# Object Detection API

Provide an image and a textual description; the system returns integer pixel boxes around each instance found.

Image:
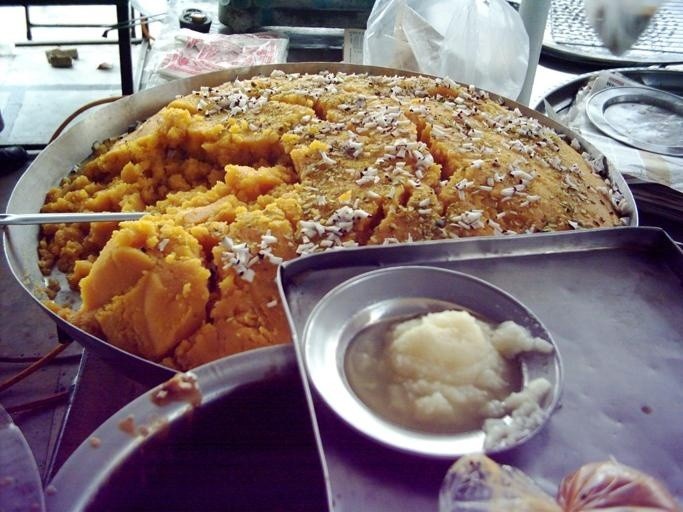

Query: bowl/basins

[42,346,316,512]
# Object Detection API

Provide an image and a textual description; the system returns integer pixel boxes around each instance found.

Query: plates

[303,264,561,458]
[586,86,683,157]
[2,60,639,373]
